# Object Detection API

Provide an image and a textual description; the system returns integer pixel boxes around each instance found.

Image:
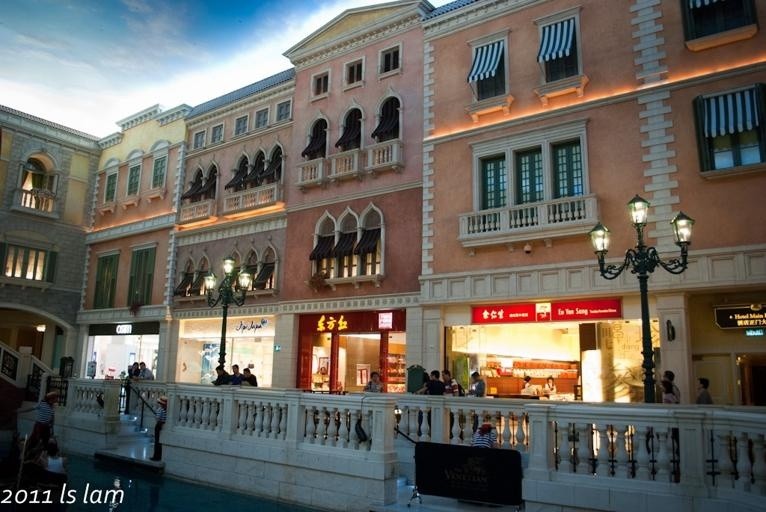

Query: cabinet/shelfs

[389,354,407,384]
[481,354,578,394]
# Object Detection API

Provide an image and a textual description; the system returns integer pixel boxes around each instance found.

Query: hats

[158,396,168,405]
[46,392,58,401]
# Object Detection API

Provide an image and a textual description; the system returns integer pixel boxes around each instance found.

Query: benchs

[407,441,526,512]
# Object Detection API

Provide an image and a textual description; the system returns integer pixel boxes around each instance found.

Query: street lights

[586,194,696,404]
[201,254,253,370]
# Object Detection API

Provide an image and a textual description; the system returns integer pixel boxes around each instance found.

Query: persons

[241,367,258,387]
[363,371,385,393]
[694,377,714,405]
[211,365,231,386]
[659,381,679,404]
[469,423,498,449]
[132,362,155,381]
[468,371,485,433]
[124,362,141,415]
[228,364,245,385]
[545,377,557,394]
[411,368,465,442]
[149,395,168,462]
[662,371,679,396]
[521,376,531,389]
[16,390,59,464]
